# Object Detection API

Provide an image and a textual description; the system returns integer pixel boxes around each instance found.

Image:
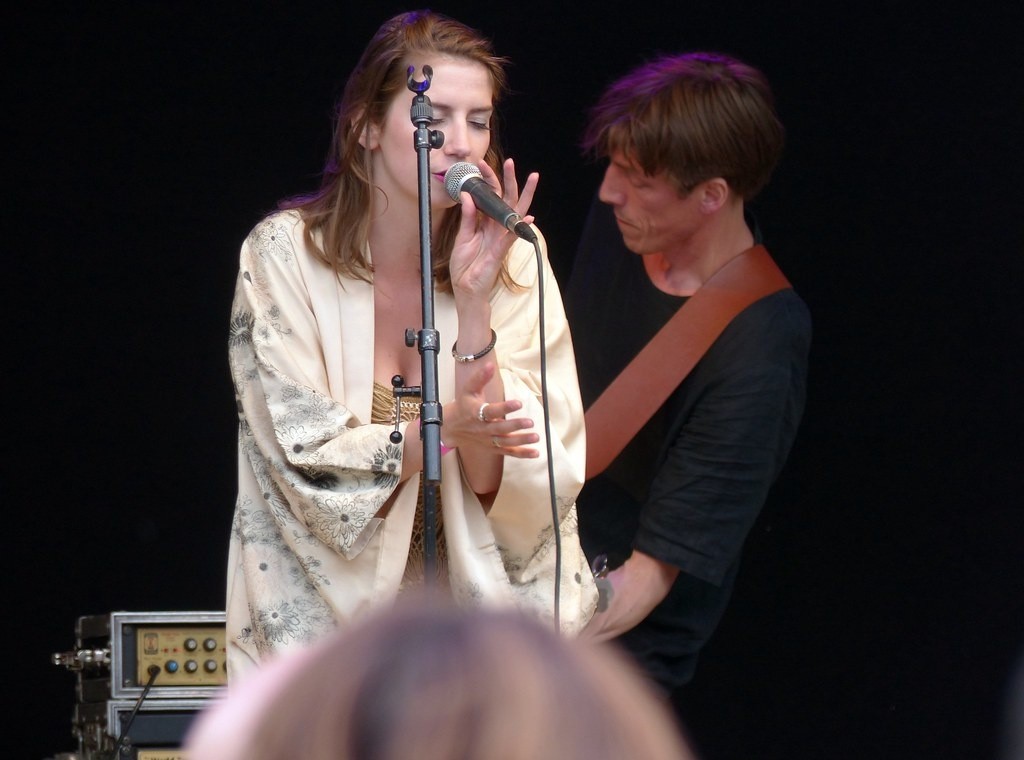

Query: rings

[478,403,497,424]
[491,436,503,448]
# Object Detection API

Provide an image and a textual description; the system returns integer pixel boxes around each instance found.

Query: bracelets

[451,328,497,363]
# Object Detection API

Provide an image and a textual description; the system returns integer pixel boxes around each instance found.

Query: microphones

[443,163,536,243]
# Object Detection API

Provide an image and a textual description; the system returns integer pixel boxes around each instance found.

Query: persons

[225,11,598,703]
[560,53,814,706]
[186,593,696,759]
